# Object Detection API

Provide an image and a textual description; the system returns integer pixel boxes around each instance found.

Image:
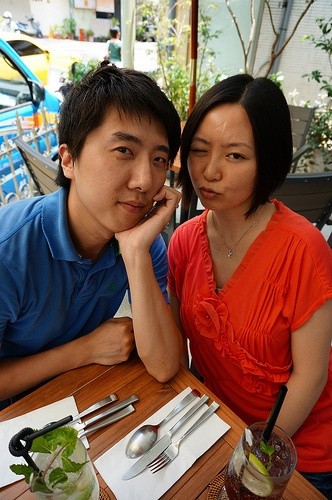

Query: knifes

[75,394,139,431]
[121,394,209,481]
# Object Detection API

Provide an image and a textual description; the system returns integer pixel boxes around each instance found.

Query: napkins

[93,387,231,500]
[0,396,90,489]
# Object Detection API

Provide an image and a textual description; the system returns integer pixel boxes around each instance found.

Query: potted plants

[63,18,76,40]
[86,30,94,42]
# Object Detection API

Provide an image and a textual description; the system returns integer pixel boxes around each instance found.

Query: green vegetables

[259,441,275,462]
[9,426,93,500]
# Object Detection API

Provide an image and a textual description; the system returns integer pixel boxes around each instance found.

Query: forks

[147,400,219,476]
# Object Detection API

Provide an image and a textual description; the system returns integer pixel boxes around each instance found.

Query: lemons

[234,450,273,497]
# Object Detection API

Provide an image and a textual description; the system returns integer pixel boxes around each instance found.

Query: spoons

[125,389,201,458]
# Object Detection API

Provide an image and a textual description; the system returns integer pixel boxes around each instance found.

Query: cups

[28,437,99,500]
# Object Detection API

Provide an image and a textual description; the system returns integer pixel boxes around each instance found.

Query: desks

[0,335,325,500]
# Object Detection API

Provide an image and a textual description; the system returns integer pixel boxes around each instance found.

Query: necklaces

[206,199,264,256]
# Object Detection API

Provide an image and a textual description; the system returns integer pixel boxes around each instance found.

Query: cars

[0,34,64,207]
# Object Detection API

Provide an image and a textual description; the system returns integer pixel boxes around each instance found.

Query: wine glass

[219,421,298,500]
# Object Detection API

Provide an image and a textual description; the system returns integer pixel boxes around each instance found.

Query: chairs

[15,141,63,198]
[282,170,332,231]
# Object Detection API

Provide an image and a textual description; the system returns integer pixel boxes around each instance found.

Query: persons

[108,25,122,71]
[0,64,182,415]
[0,9,20,34]
[167,73,332,500]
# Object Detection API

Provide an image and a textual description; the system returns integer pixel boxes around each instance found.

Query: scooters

[14,16,43,38]
